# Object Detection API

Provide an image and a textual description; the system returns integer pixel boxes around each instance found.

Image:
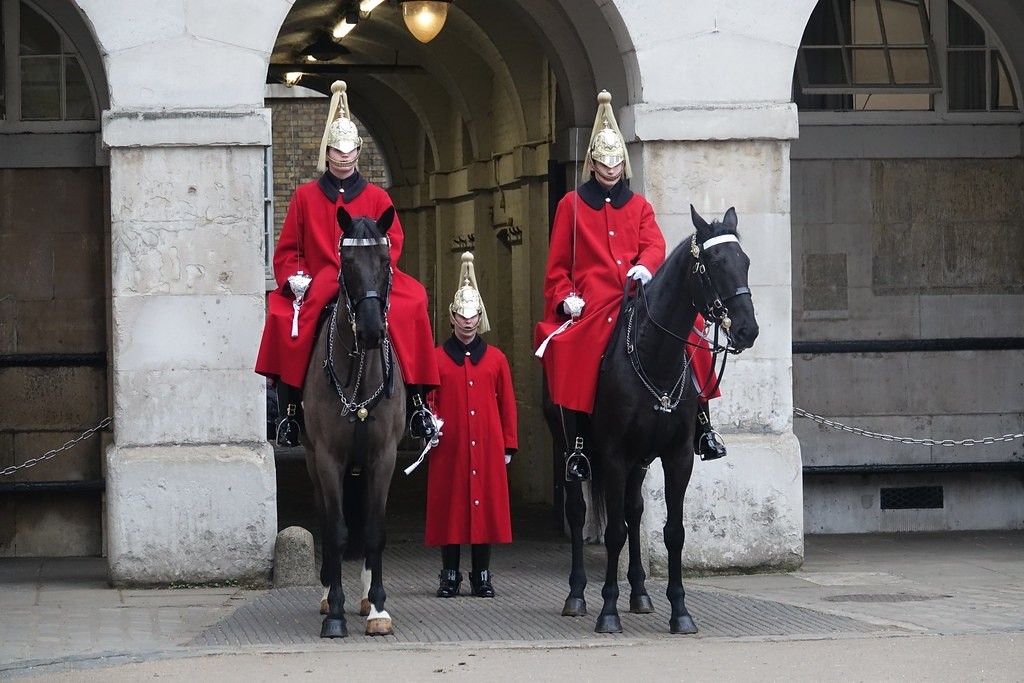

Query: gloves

[505,454,512,464]
[564,294,585,317]
[430,430,442,447]
[626,264,652,286]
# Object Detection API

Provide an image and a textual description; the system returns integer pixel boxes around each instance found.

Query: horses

[541,203,760,635]
[302,205,408,638]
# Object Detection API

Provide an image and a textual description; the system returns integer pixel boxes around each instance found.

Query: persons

[254,80,441,447]
[532,88,727,482]
[428,251,516,598]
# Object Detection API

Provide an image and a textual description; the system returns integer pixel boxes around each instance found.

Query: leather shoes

[278,413,298,445]
[694,425,727,459]
[437,570,464,597]
[408,401,434,435]
[471,570,495,597]
[563,441,591,478]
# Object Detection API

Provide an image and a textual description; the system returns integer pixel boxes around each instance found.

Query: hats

[448,252,491,334]
[581,88,632,184]
[317,80,362,175]
